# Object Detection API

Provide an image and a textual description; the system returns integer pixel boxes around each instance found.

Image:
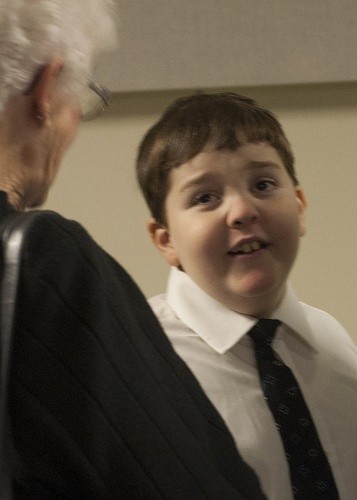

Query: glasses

[80,79,113,122]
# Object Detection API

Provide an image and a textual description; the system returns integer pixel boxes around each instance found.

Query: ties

[247,319,342,500]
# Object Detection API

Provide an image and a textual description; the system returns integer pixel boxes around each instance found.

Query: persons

[0,1,268,500]
[136,91,357,500]
